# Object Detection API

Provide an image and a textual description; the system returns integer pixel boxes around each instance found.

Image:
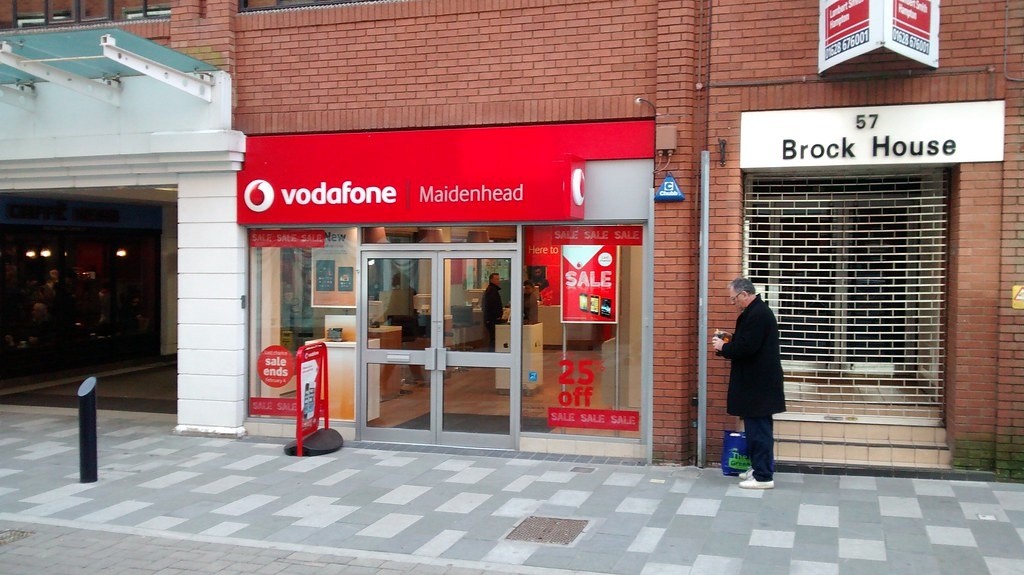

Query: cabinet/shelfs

[304,305,603,423]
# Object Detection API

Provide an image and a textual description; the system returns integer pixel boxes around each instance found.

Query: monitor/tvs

[369,300,384,329]
[467,291,483,308]
[413,296,432,314]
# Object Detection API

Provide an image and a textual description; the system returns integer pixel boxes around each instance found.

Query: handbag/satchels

[721,430,775,476]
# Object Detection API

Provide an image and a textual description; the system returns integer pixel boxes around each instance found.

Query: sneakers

[738,476,774,489]
[738,466,755,478]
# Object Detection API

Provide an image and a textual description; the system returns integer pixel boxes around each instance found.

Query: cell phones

[579,293,612,318]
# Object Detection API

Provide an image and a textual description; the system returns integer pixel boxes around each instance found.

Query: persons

[524,280,538,325]
[482,273,503,352]
[712,278,787,489]
[533,267,546,286]
[537,279,553,306]
[370,273,425,399]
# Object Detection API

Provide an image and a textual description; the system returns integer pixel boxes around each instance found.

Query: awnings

[0,30,219,81]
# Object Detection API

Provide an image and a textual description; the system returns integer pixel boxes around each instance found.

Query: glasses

[731,293,741,302]
